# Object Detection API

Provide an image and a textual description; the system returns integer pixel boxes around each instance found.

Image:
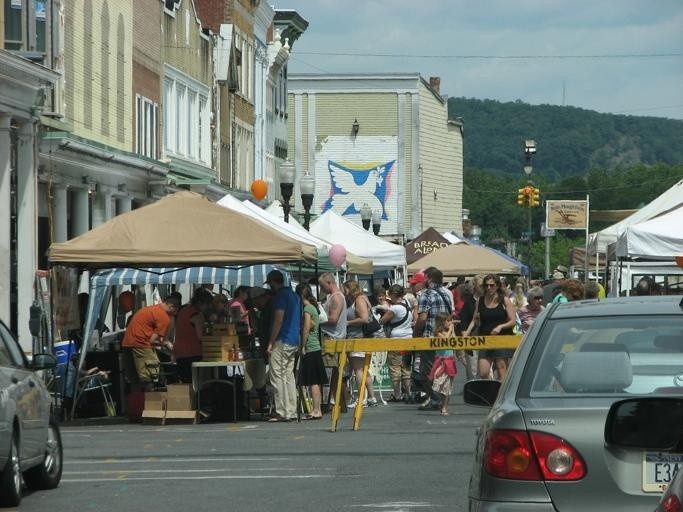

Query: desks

[191,358,270,423]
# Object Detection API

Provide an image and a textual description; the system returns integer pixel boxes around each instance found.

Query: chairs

[67,362,117,420]
[559,351,634,393]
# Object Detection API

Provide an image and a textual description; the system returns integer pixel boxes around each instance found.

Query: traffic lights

[522,188,531,209]
[533,188,540,208]
[517,186,526,208]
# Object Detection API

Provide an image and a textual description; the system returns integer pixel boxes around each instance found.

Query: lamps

[352,116,360,139]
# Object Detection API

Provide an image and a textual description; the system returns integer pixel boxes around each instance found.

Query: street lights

[280,152,314,290]
[520,137,539,282]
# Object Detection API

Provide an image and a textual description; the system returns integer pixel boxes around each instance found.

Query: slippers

[439,409,448,416]
[387,392,428,402]
[267,414,292,423]
[300,413,322,421]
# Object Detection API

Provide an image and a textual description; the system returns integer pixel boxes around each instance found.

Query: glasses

[534,295,545,301]
[484,283,497,288]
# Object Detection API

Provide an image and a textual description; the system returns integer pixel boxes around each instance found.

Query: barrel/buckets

[43,339,78,393]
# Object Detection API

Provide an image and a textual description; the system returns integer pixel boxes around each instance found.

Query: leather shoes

[419,401,441,411]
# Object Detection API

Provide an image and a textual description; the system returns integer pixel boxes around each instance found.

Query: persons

[637,275,667,296]
[65,291,112,388]
[122,268,605,423]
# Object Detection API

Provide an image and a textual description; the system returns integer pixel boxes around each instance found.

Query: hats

[409,274,424,284]
[263,270,284,284]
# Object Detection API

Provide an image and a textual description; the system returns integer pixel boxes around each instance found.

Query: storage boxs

[144,383,192,411]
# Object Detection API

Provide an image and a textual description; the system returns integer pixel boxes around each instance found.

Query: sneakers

[347,398,370,410]
[366,397,379,407]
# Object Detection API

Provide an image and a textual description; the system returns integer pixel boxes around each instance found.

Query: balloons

[327,245,346,267]
[250,180,268,202]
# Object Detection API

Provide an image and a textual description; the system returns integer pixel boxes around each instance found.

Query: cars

[1,321,63,507]
[461,291,683,512]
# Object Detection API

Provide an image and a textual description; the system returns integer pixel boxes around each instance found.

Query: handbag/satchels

[317,303,329,325]
[362,317,381,337]
